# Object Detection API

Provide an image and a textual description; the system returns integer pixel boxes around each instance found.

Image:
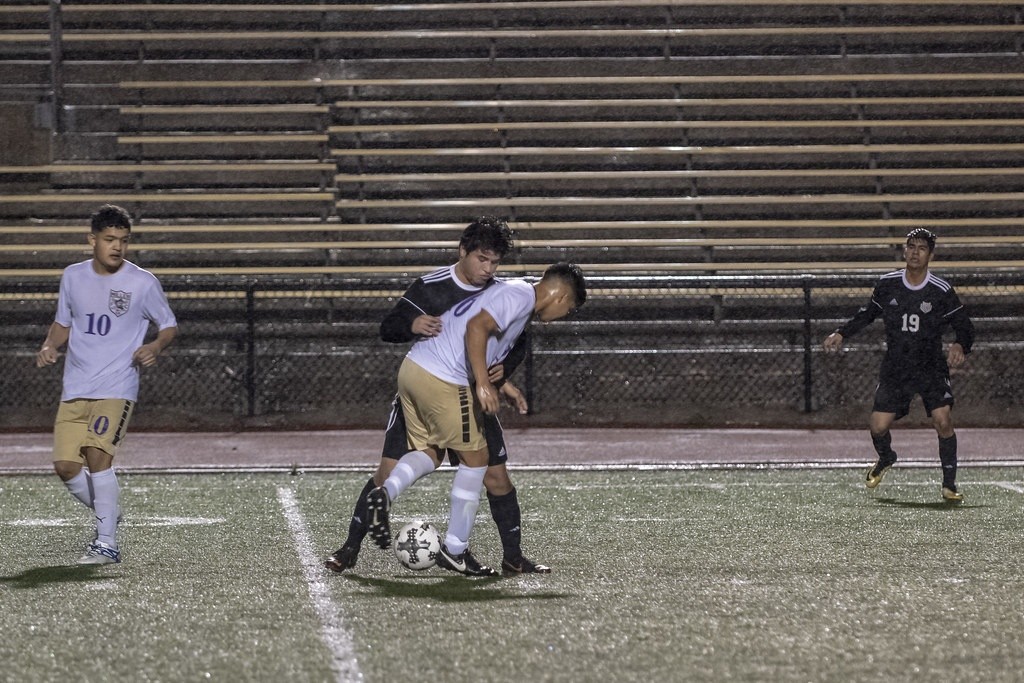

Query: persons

[323,212,552,576]
[365,263,587,577]
[34,204,181,566]
[822,228,977,501]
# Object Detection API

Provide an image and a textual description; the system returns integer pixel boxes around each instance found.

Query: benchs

[1,1,1024,419]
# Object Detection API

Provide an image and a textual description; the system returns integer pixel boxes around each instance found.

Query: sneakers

[368,486,392,551]
[436,541,498,576]
[326,543,360,572]
[502,556,552,574]
[76,541,121,565]
[868,449,897,488]
[942,485,962,500]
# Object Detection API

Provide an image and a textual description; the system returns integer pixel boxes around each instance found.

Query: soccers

[393,518,441,571]
